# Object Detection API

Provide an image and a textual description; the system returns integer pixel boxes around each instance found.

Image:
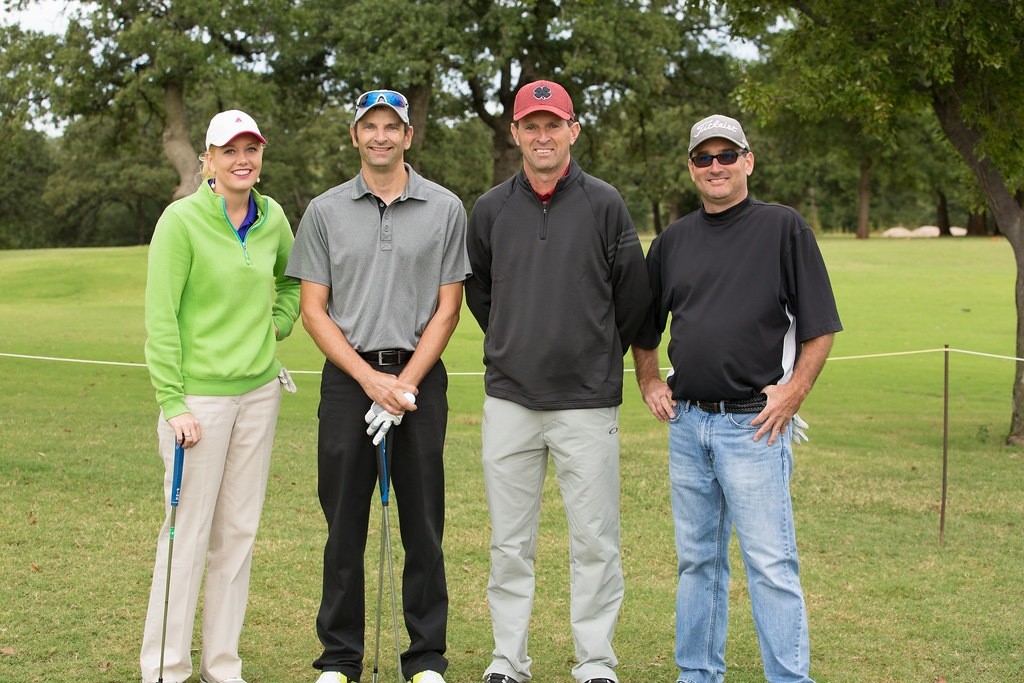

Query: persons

[282,88,473,682]
[139,109,302,683]
[462,78,653,682]
[628,112,847,682]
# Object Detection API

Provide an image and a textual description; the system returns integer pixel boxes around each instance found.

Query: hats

[687,114,750,154]
[513,79,573,122]
[352,88,412,126]
[205,109,266,147]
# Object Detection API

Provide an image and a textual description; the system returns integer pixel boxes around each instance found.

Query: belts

[357,350,417,367]
[689,395,768,414]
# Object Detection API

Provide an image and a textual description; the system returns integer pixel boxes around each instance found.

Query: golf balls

[404,392,416,405]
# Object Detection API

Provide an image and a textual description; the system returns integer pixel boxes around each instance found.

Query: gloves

[364,393,418,445]
[278,367,298,394]
[792,413,810,445]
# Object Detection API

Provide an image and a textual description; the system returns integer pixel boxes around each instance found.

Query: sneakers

[407,670,447,683]
[484,673,519,683]
[314,671,358,683]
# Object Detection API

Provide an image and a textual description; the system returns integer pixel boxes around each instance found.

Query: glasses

[690,148,745,168]
[356,90,407,108]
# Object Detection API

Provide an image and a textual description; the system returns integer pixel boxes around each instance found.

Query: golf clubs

[156,433,185,683]
[373,425,395,683]
[374,427,404,683]
